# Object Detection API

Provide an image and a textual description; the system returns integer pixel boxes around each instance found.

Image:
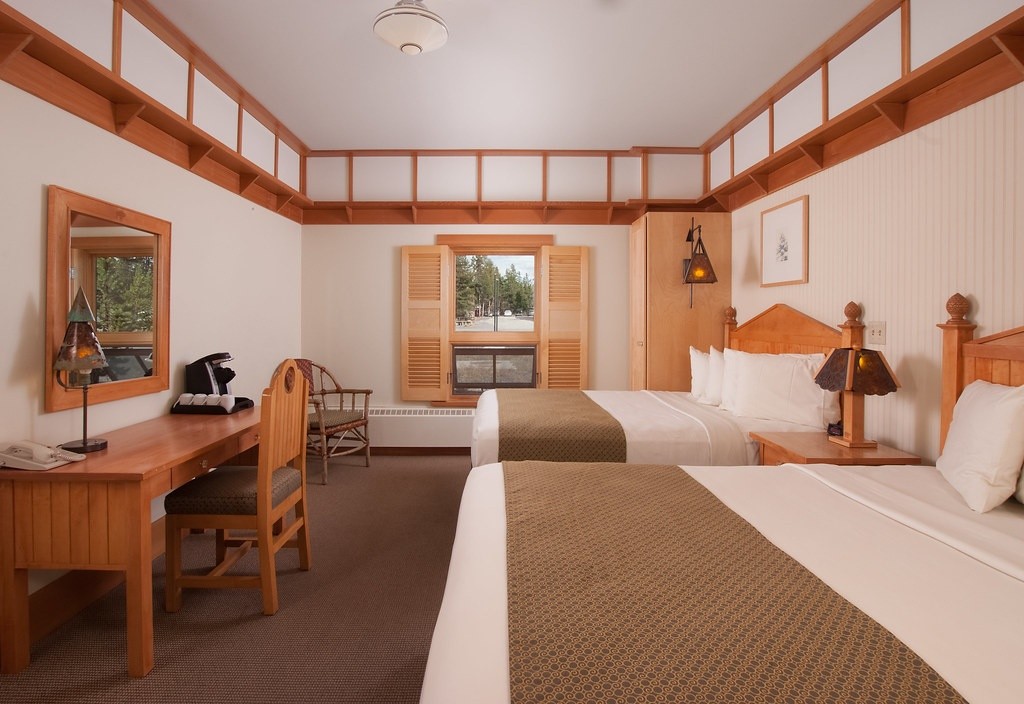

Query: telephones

[0,440,78,471]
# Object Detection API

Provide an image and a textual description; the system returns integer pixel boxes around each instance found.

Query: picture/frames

[760,194,809,287]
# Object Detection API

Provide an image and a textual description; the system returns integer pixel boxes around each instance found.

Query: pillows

[935,378,1024,515]
[685,345,842,430]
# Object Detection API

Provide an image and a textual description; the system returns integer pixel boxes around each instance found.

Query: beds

[417,293,1024,703]
[471,301,866,466]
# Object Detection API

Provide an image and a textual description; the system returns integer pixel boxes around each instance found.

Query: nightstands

[749,430,921,466]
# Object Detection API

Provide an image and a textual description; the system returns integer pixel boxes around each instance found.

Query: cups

[188,394,207,405]
[201,394,220,405]
[173,393,194,408]
[216,393,235,413]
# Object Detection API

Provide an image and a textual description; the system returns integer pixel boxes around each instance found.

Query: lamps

[51,288,112,453]
[812,348,901,448]
[682,218,719,308]
[373,0,450,56]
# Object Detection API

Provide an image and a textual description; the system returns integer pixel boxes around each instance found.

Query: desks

[0,406,287,676]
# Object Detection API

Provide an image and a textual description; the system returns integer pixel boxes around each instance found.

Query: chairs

[274,358,374,486]
[165,358,314,616]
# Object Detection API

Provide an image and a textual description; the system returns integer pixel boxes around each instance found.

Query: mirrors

[43,184,172,410]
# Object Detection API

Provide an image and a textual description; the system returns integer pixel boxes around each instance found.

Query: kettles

[185,352,236,395]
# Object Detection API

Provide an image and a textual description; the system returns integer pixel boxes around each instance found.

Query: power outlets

[867,321,885,345]
